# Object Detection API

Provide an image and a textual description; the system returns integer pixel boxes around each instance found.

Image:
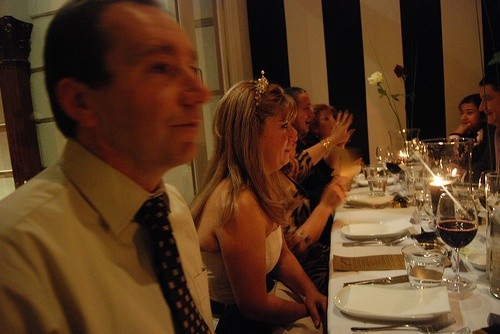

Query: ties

[132,193,212,334]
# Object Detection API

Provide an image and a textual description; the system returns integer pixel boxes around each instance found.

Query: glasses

[97,59,204,91]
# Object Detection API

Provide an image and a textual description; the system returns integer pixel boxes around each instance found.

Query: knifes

[343,275,409,288]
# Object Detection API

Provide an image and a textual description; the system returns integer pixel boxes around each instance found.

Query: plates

[345,194,394,206]
[466,248,487,270]
[334,285,442,322]
[357,177,396,186]
[341,222,405,239]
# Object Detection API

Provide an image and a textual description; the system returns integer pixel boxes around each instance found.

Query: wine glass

[375,145,392,176]
[386,155,404,185]
[435,193,480,294]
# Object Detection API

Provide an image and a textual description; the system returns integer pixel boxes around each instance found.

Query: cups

[368,176,388,196]
[388,128,420,166]
[478,171,491,208]
[484,170,500,299]
[403,243,448,288]
[419,138,472,204]
[362,164,376,181]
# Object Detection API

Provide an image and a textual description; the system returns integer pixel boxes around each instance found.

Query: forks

[455,326,471,334]
[350,317,456,334]
[342,233,408,247]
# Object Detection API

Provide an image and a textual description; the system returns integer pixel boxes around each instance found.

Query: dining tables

[326,177,500,334]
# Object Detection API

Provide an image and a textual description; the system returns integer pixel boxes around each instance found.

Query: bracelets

[320,137,335,153]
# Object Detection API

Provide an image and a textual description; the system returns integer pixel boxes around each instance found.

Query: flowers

[367,64,411,149]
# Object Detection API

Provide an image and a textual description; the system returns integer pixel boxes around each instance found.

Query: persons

[188,69,328,334]
[280,85,367,300]
[0,0,214,333]
[448,67,500,194]
